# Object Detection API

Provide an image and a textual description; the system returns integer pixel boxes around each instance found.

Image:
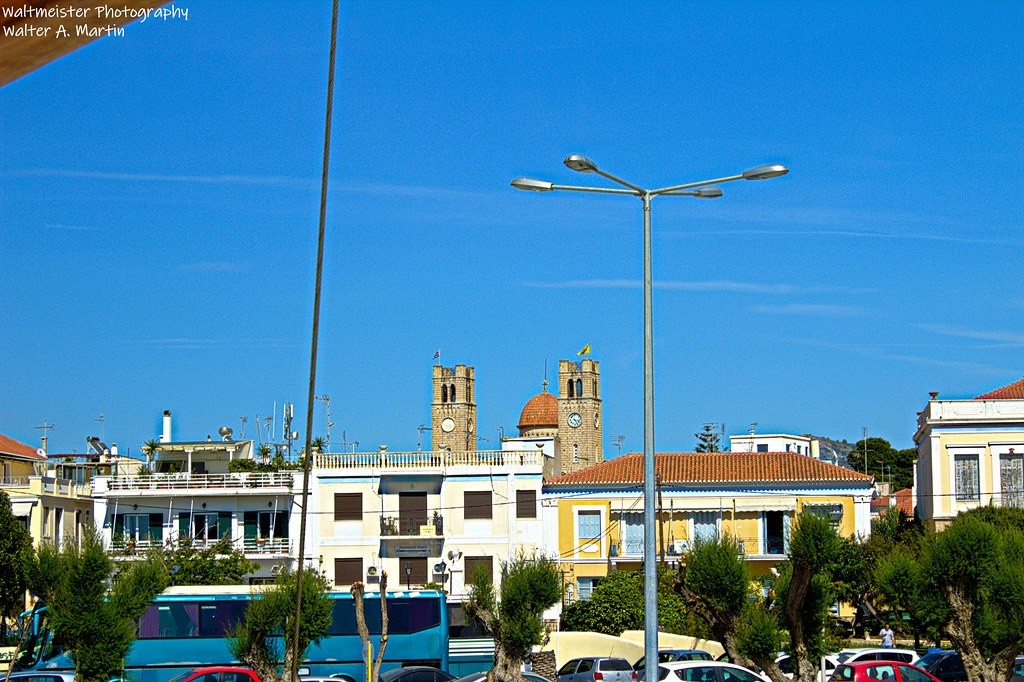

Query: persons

[852,607,865,638]
[879,623,896,649]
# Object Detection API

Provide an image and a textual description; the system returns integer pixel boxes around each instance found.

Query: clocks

[593,412,599,429]
[568,412,582,428]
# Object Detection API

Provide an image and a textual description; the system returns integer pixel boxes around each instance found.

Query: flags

[577,344,590,356]
[433,351,439,360]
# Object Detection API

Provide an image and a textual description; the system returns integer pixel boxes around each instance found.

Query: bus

[5,584,448,682]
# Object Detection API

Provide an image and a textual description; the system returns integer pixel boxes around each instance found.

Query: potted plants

[137,463,273,487]
[128,529,289,553]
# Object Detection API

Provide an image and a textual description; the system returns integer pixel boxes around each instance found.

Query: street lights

[510,152,788,682]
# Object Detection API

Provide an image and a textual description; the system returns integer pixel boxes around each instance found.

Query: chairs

[706,670,714,682]
[580,665,589,672]
[678,656,687,661]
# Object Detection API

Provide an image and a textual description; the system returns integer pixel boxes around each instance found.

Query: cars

[0,650,840,682]
[828,659,941,682]
[844,649,919,665]
[913,650,966,682]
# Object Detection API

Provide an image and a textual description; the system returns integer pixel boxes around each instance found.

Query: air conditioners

[431,561,449,574]
[367,566,383,576]
